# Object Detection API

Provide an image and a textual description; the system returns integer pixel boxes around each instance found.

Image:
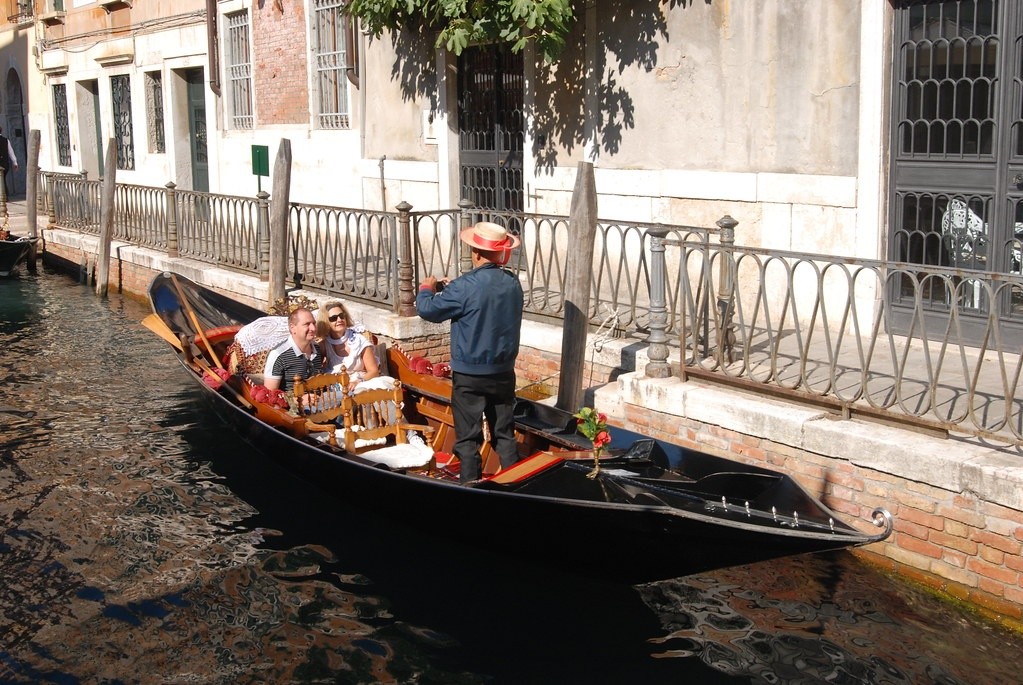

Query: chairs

[292,366,437,479]
[941,198,1023,310]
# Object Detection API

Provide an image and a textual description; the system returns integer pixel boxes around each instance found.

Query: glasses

[328,312,345,322]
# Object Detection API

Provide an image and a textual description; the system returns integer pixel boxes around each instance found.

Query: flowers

[573,406,612,479]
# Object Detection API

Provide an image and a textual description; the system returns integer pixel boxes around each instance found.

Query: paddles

[141,312,254,410]
[170,273,224,370]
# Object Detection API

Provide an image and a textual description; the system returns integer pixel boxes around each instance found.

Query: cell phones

[436,282,444,292]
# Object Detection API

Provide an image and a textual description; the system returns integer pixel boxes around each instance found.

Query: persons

[316,301,424,446]
[416,222,523,483]
[264,308,357,428]
[0,126,19,202]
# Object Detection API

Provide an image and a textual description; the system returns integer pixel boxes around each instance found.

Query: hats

[460,222,520,251]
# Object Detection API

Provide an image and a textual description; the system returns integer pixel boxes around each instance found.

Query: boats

[0,227,42,277]
[144,266,898,605]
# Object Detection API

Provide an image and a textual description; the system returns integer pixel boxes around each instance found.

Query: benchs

[223,314,394,412]
[194,325,243,353]
